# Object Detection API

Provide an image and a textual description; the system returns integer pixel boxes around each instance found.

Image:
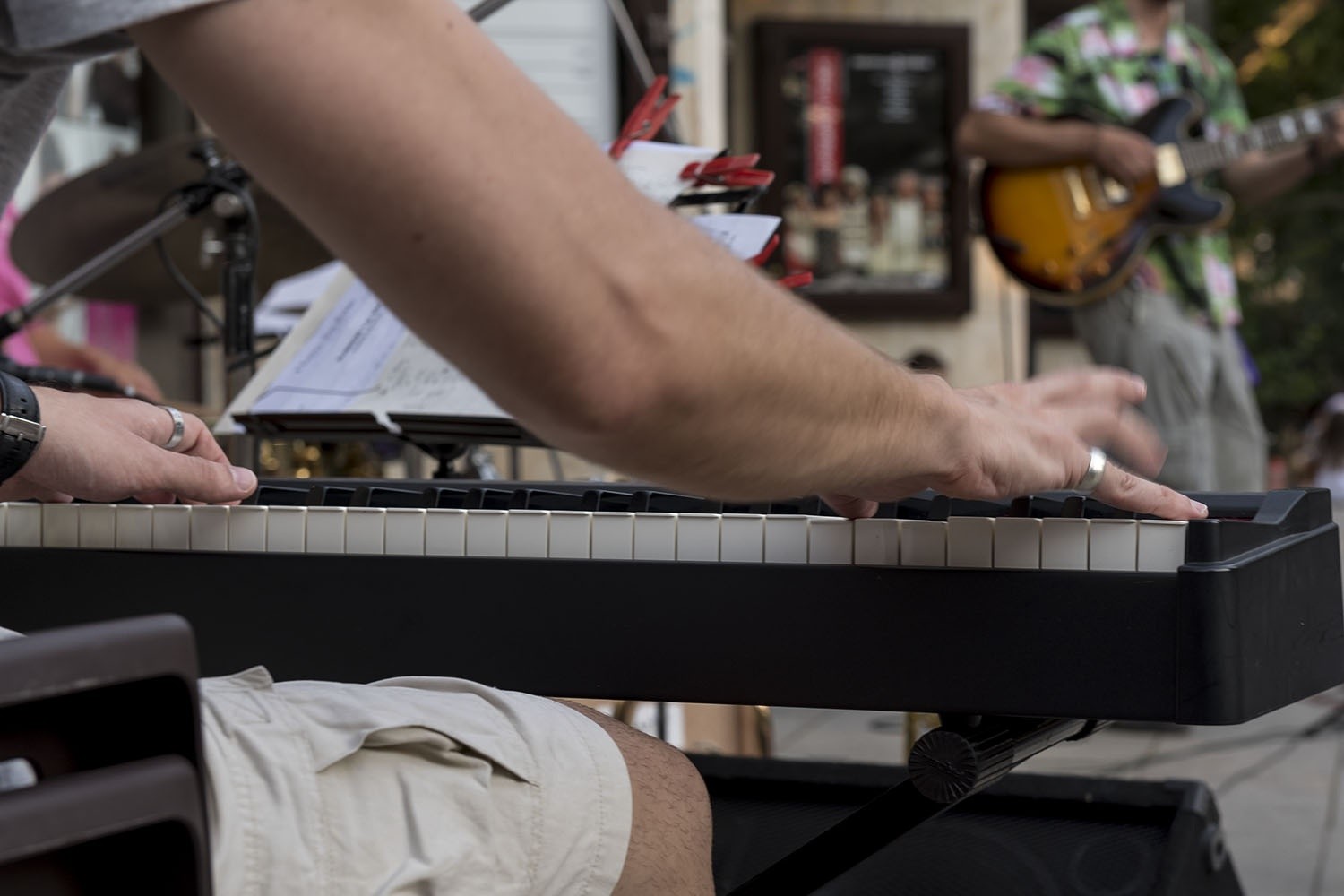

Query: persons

[958,0,1344,494]
[0,0,1207,896]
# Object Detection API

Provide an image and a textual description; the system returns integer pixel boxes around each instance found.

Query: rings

[1074,445,1107,494]
[158,406,185,449]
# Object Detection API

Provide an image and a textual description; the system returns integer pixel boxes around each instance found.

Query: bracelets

[0,371,47,487]
[1307,139,1323,169]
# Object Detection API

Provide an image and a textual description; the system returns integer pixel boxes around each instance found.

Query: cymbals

[9,134,342,307]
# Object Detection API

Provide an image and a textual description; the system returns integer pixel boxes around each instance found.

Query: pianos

[0,477,1344,896]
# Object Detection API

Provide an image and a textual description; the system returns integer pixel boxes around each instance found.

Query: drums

[201,414,379,481]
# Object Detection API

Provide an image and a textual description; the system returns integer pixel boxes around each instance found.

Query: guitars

[968,95,1344,307]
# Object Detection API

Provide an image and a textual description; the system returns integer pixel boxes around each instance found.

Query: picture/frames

[750,21,973,322]
